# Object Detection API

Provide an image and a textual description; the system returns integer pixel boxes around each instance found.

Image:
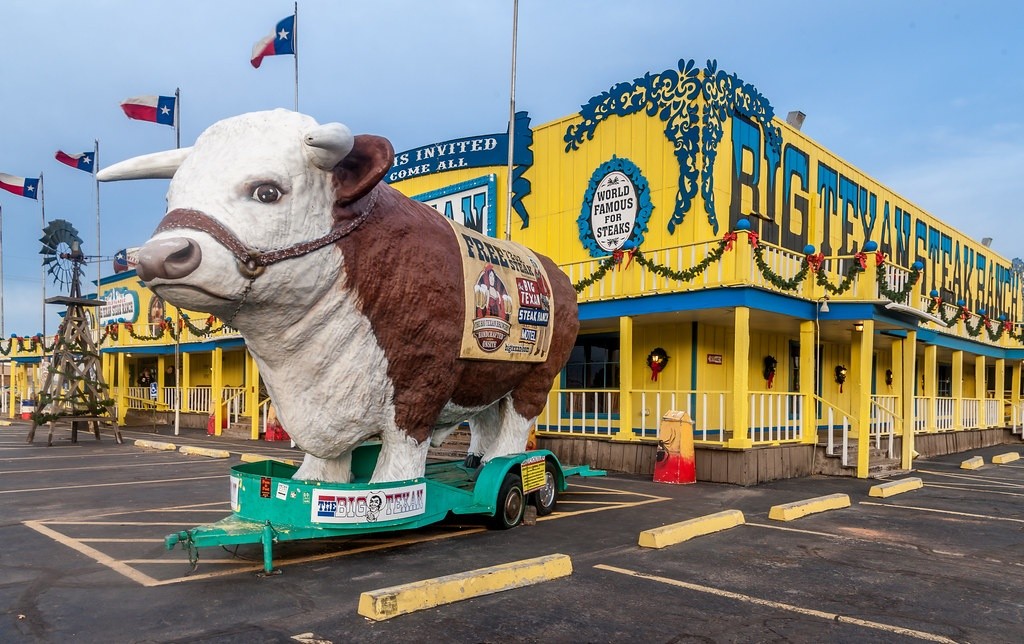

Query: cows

[96,107,580,483]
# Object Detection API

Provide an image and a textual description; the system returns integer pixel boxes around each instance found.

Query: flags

[0,172,39,200]
[251,15,295,70]
[55,150,94,173]
[120,95,175,126]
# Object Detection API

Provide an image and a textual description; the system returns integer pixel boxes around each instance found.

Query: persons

[136,368,155,399]
[164,366,176,409]
